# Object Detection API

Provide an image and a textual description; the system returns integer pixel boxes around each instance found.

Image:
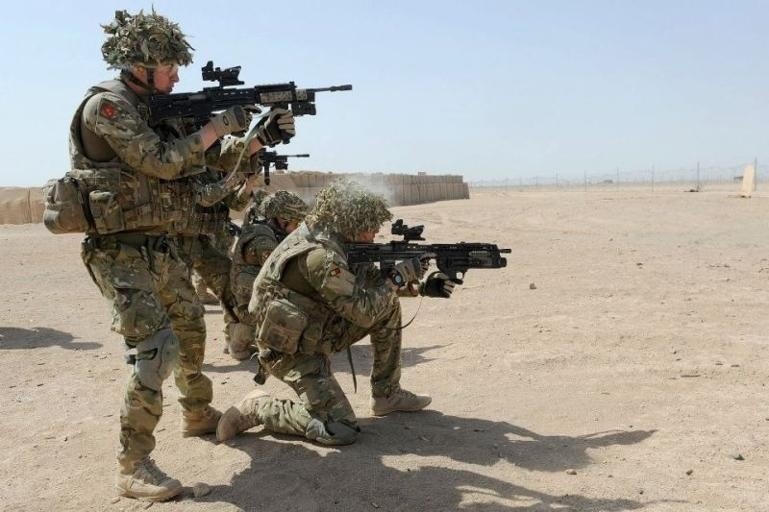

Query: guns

[146,60,353,147]
[258,149,310,183]
[342,219,511,281]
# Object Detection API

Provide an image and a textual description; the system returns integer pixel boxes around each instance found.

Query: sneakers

[183,405,223,438]
[195,287,218,304]
[117,457,183,502]
[370,389,432,416]
[215,389,270,442]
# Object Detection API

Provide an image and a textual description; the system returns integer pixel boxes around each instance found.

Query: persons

[68,10,294,502]
[194,271,218,304]
[216,176,454,445]
[192,173,270,354]
[227,191,310,361]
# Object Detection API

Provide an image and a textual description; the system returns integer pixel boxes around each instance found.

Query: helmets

[310,177,393,233]
[254,189,309,223]
[101,10,195,69]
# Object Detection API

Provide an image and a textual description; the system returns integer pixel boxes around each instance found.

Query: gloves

[418,272,462,297]
[393,253,430,282]
[257,108,295,145]
[211,103,261,137]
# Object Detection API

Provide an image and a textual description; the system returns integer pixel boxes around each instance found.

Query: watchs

[388,272,405,286]
